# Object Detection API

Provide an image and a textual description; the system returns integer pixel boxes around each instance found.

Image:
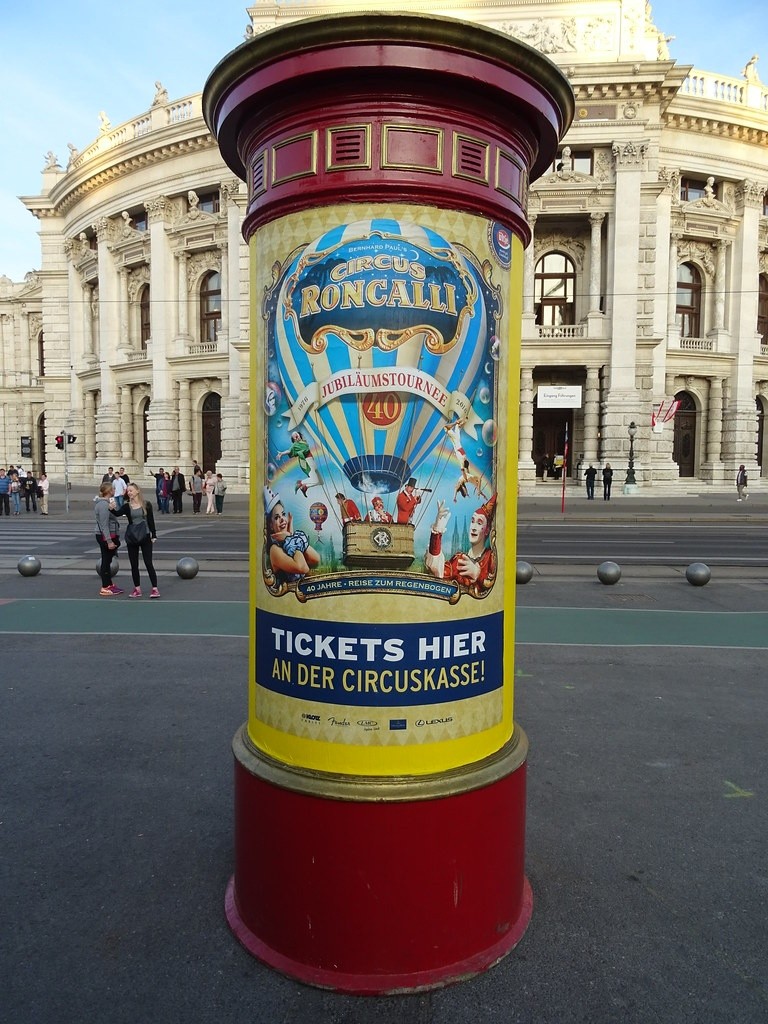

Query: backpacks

[555,455,563,468]
[185,476,196,496]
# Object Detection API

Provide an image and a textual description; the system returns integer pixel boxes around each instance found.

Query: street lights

[623,421,637,484]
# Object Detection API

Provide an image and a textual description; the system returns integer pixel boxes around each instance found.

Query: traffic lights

[66,434,78,444]
[53,435,64,451]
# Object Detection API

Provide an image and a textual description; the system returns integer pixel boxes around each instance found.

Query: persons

[152,82,169,106]
[151,459,227,515]
[584,463,597,500]
[0,465,49,516]
[102,467,130,517]
[80,232,88,245]
[263,417,498,593]
[94,482,125,596]
[67,143,79,160]
[542,451,562,482]
[188,191,199,206]
[595,152,610,181]
[99,112,111,133]
[704,177,715,192]
[562,147,571,163]
[503,4,675,62]
[725,187,735,207]
[108,483,160,597]
[43,151,62,170]
[602,463,613,501]
[736,465,749,502]
[740,55,759,84]
[122,211,133,226]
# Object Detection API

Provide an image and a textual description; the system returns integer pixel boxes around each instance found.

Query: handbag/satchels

[124,521,148,547]
[103,534,122,552]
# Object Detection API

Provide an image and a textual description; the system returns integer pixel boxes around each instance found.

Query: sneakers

[736,499,742,501]
[150,587,161,598]
[745,494,749,500]
[128,586,143,598]
[99,584,124,595]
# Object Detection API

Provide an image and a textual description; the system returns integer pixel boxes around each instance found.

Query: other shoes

[122,514,127,517]
[173,511,177,514]
[608,498,610,500]
[157,509,161,511]
[162,512,164,514]
[13,512,20,515]
[0,514,2,516]
[604,498,606,500]
[542,481,547,482]
[194,512,202,515]
[40,513,48,515]
[6,514,9,516]
[587,497,594,500]
[179,510,182,513]
[166,512,170,514]
[206,512,213,515]
[215,513,222,515]
[553,477,559,480]
